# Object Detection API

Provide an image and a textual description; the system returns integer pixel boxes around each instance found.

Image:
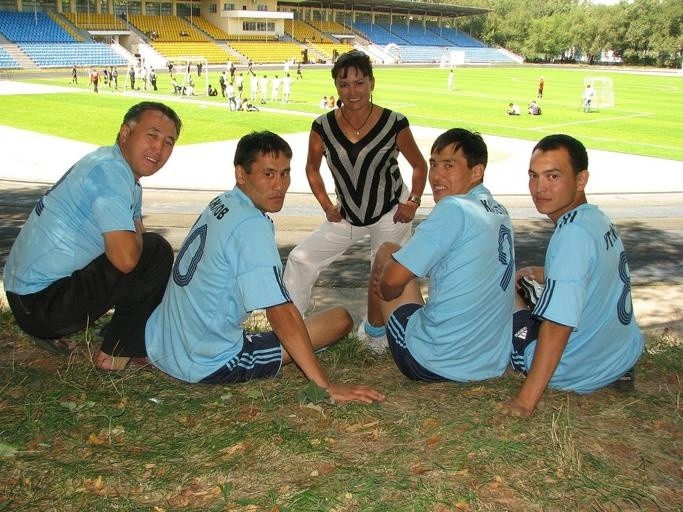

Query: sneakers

[615,368,634,391]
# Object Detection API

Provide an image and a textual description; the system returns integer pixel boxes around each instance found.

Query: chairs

[0,11,523,70]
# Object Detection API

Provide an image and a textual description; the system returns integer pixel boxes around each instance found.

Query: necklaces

[339,104,374,136]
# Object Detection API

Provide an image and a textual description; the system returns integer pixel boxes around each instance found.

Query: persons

[221,60,293,112]
[180,31,190,37]
[363,128,517,383]
[448,70,453,90]
[91,69,100,93]
[173,76,194,96]
[536,76,545,99]
[168,64,173,77]
[208,84,216,96]
[142,128,386,405]
[72,65,78,84]
[281,45,428,355]
[3,100,183,372]
[146,31,159,42]
[526,100,542,116]
[129,66,157,90]
[496,133,645,420]
[284,60,303,79]
[320,96,335,110]
[582,84,595,112]
[104,66,118,90]
[121,11,126,17]
[506,102,522,116]
[197,63,202,77]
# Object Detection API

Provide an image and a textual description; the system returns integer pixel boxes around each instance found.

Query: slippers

[114,356,154,372]
[38,337,71,355]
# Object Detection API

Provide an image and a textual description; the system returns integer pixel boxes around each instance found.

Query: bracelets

[407,194,423,207]
